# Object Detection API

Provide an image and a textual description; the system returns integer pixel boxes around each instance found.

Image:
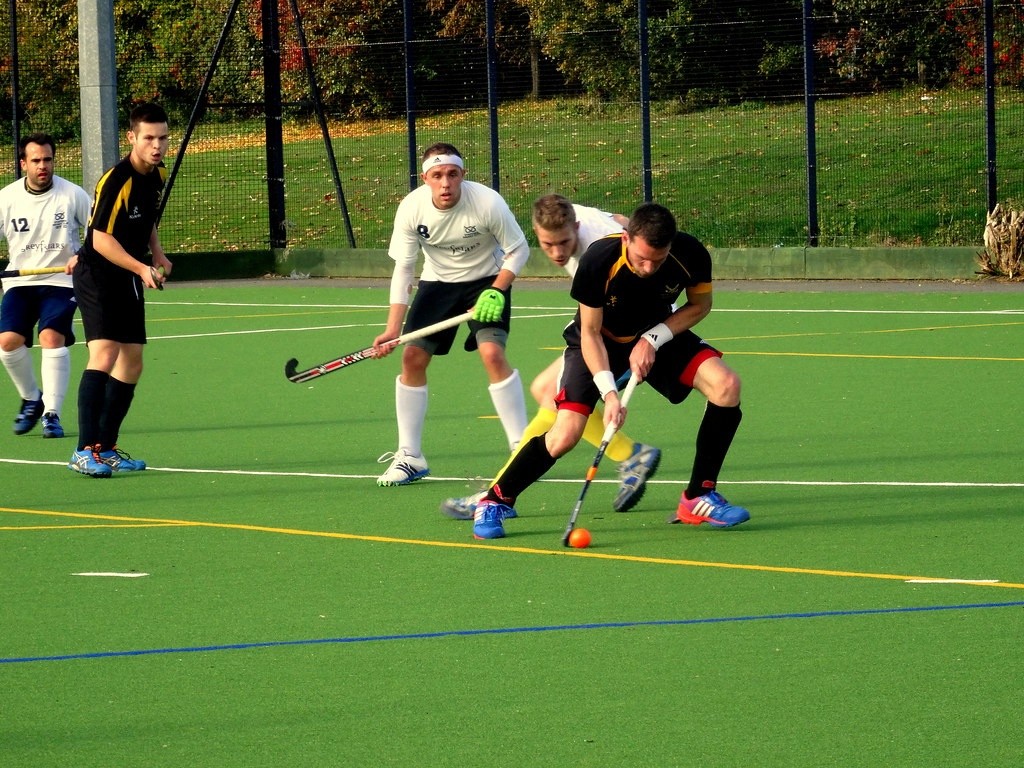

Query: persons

[471,203,751,539]
[0,132,93,437]
[66,102,173,475]
[375,142,532,486]
[442,192,661,520]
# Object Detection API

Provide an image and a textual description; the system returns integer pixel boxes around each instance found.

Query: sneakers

[41,412,64,438]
[473,495,511,539]
[377,449,431,486]
[614,442,661,512]
[67,443,112,477]
[14,389,45,435]
[677,490,750,528]
[99,444,146,472]
[441,489,518,519]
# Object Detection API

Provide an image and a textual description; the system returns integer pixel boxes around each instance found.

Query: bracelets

[593,370,619,400]
[640,323,674,351]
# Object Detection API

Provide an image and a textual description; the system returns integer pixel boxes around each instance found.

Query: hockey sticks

[561,370,640,547]
[284,311,475,385]
[0,265,67,279]
[149,265,166,292]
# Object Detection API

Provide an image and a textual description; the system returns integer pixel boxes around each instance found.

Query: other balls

[568,528,592,550]
